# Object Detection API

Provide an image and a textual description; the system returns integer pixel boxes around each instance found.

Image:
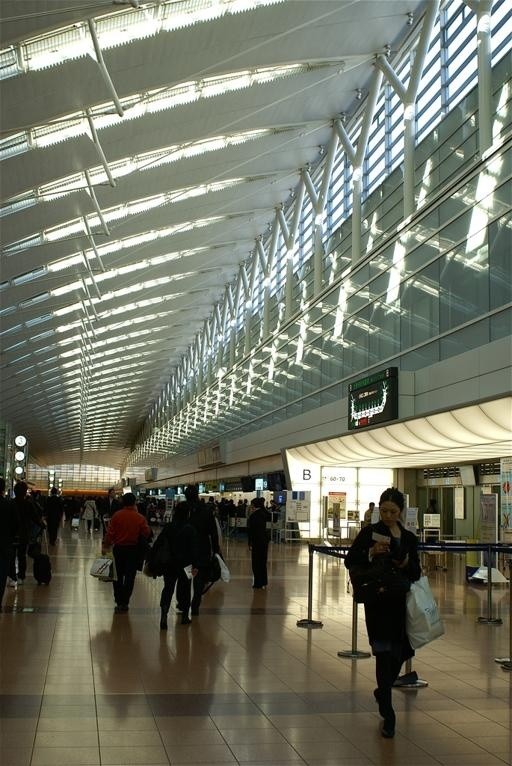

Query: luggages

[93,516,101,531]
[33,529,51,586]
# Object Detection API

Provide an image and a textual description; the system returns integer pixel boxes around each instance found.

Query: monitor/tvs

[255,478,263,490]
[149,483,205,495]
[220,482,225,492]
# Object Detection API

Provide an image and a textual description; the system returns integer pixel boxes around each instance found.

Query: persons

[1,481,63,600]
[364,502,375,522]
[200,497,282,550]
[62,494,109,534]
[146,486,222,628]
[247,497,268,590]
[99,487,177,554]
[102,492,151,615]
[346,488,435,742]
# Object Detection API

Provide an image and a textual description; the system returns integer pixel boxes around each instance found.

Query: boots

[159,604,169,629]
[179,608,192,625]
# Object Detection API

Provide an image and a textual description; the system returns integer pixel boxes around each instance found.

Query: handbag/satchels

[347,563,375,603]
[206,550,231,585]
[89,552,118,583]
[27,541,42,560]
[406,570,446,650]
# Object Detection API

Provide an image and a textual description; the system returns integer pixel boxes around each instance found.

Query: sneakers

[380,720,396,739]
